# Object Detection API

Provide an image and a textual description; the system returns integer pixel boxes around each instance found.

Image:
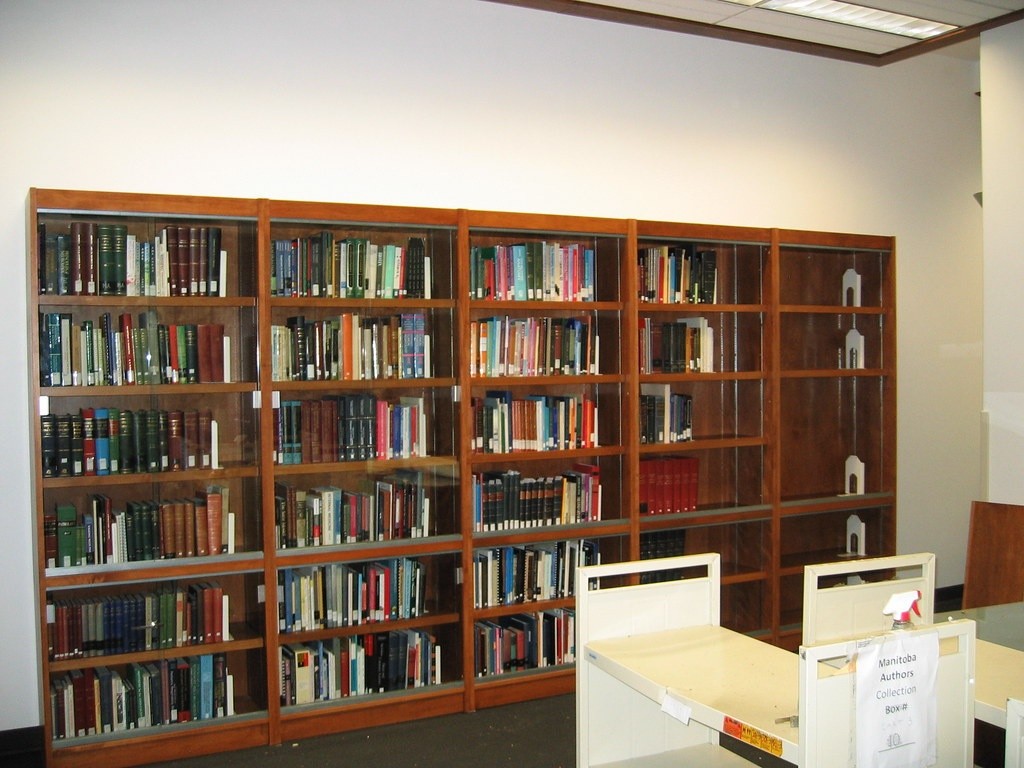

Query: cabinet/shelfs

[255,196,468,725]
[624,217,781,659]
[456,200,636,693]
[25,185,273,758]
[781,226,900,640]
[801,551,1023,768]
[576,553,976,767]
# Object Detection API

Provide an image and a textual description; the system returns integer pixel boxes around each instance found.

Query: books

[49,657,247,748]
[474,307,603,383]
[270,310,439,382]
[34,214,236,302]
[276,554,448,627]
[44,482,246,572]
[278,393,442,464]
[637,241,731,309]
[34,403,229,480]
[473,457,703,535]
[276,472,443,547]
[266,227,447,299]
[474,384,698,452]
[474,539,605,607]
[472,228,600,304]
[270,637,451,708]
[641,313,727,375]
[34,310,235,392]
[473,614,581,681]
[45,580,243,656]
